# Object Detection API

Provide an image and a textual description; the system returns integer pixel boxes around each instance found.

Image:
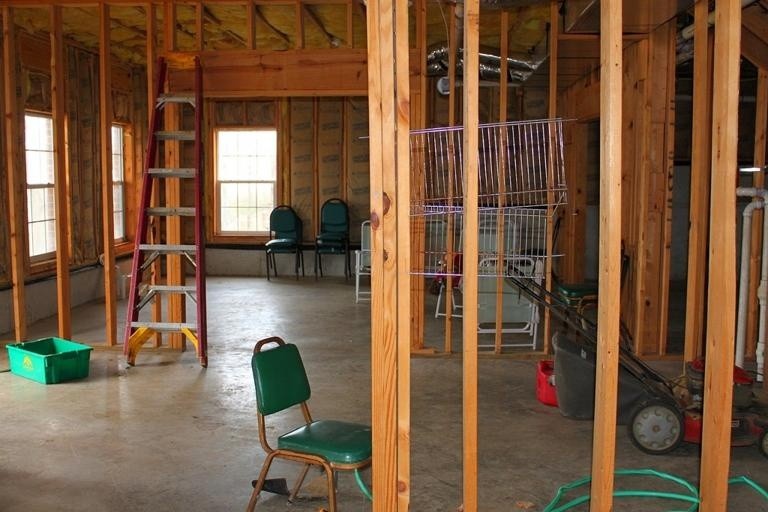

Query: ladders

[124,57,207,366]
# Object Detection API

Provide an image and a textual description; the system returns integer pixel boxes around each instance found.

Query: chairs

[264,196,370,305]
[434,218,518,321]
[247,335,371,512]
